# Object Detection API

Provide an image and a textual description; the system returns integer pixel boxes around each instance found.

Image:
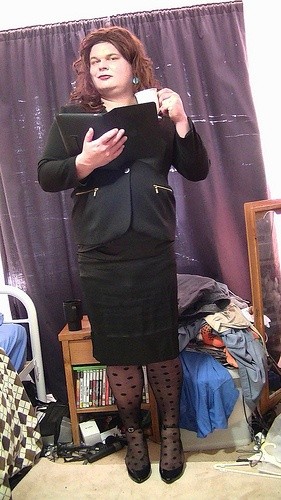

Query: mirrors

[244,196,281,415]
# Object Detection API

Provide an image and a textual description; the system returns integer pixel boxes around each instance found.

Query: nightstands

[59,314,162,446]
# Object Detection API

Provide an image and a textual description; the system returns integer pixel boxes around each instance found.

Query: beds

[0,283,47,500]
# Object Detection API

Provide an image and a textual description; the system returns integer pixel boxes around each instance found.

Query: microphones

[86,440,126,463]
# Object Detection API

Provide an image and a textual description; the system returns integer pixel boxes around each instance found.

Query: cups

[63,299,83,331]
[134,87,166,115]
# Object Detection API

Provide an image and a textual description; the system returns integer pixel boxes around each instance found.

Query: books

[53,103,161,170]
[73,365,149,409]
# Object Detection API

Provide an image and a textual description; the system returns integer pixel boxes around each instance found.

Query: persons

[38,26,211,483]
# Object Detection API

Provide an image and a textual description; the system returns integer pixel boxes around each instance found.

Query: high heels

[125,439,153,484]
[159,437,184,482]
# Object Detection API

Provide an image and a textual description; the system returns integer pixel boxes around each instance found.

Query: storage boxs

[182,364,256,453]
